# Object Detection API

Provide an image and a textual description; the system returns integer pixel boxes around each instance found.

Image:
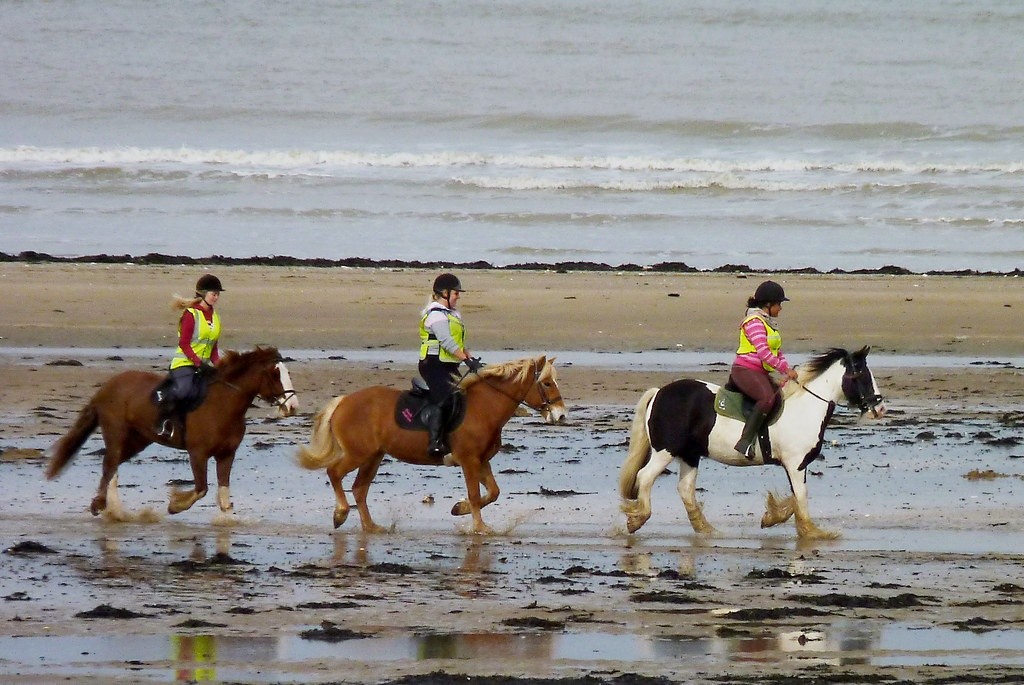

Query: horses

[44,345,299,517]
[619,344,889,540]
[296,352,566,535]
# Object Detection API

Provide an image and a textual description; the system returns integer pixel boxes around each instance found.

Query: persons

[151,274,225,434]
[731,281,798,458]
[418,273,482,452]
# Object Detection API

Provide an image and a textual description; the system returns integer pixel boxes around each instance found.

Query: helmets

[433,273,466,292]
[195,274,226,292]
[753,280,791,303]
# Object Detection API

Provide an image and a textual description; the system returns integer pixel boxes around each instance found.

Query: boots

[427,400,452,455]
[734,406,768,460]
[155,396,181,436]
